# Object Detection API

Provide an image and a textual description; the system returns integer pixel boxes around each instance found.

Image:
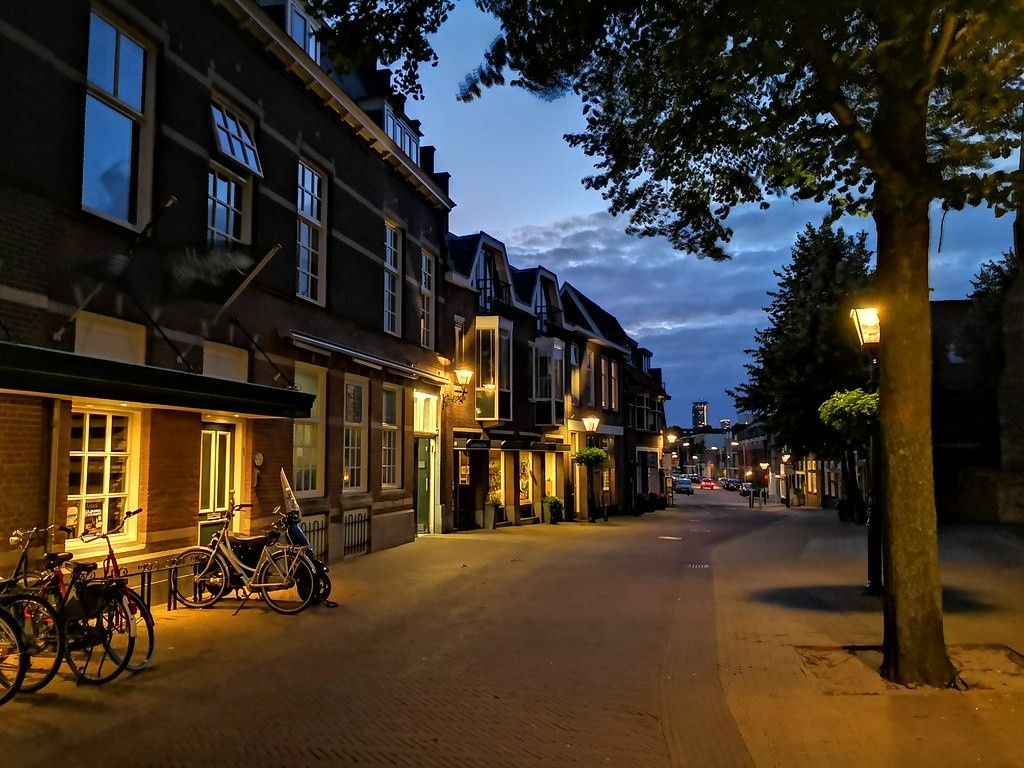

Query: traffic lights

[763,474,768,482]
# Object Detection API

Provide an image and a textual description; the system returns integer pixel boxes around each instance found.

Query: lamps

[442,362,475,410]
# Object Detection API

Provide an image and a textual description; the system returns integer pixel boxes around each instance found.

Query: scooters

[204,505,332,605]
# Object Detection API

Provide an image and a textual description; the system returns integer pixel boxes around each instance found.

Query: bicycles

[0,508,156,707]
[169,490,317,615]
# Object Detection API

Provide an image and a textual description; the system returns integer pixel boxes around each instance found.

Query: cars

[701,478,714,489]
[671,471,699,483]
[717,477,744,491]
[676,479,694,495]
[739,482,758,497]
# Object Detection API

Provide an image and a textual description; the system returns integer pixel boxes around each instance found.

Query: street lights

[781,454,791,510]
[849,306,883,597]
[760,463,770,504]
[580,413,601,524]
